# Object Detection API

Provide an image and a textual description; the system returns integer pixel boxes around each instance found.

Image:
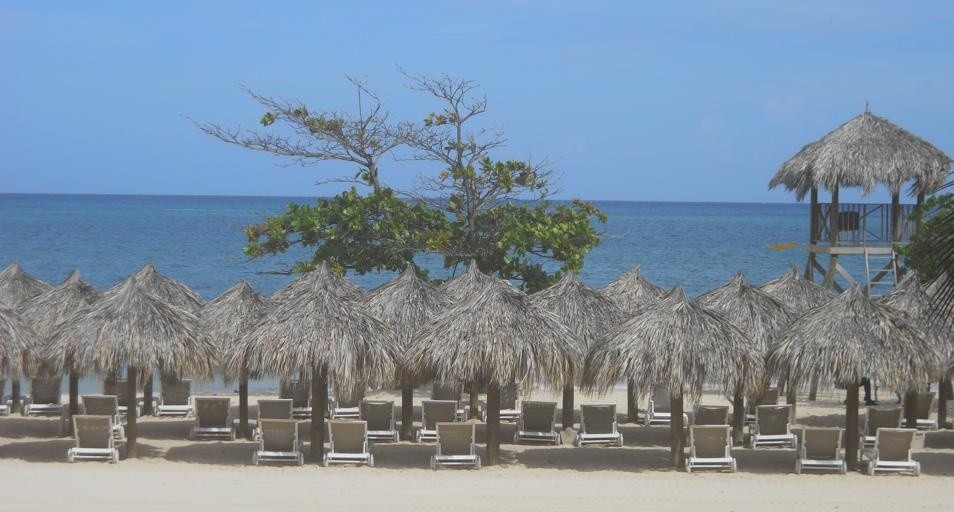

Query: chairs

[429,422,481,471]
[866,425,921,477]
[796,427,848,473]
[856,407,903,465]
[685,425,737,473]
[252,419,305,465]
[825,211,858,241]
[66,414,120,462]
[321,420,375,468]
[0,371,935,450]
